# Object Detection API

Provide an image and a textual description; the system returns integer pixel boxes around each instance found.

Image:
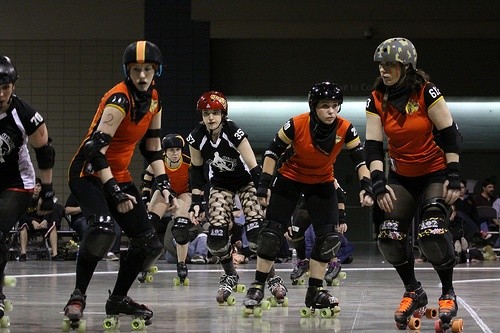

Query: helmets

[162,134,185,149]
[197,91,228,114]
[122,40,164,80]
[373,37,417,70]
[0,54,17,84]
[307,82,343,110]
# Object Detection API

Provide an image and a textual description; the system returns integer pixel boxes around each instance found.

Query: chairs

[477,207,499,251]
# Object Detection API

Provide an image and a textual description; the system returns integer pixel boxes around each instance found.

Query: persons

[63,41,179,330]
[65,192,121,260]
[16,178,65,262]
[365,38,463,333]
[243,80,374,317]
[0,54,55,328]
[449,178,500,255]
[167,192,256,264]
[186,91,288,307]
[290,176,347,286]
[137,132,205,285]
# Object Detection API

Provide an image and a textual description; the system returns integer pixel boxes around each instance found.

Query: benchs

[6,229,128,261]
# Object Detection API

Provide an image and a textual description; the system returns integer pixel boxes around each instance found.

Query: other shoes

[19,254,26,260]
[106,253,119,260]
[190,254,206,264]
[205,255,212,264]
[452,245,497,263]
[275,257,284,263]
[51,254,64,261]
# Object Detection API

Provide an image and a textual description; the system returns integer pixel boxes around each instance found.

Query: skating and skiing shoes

[61,289,87,333]
[216,272,245,306]
[266,275,289,307]
[173,262,190,285]
[242,281,270,318]
[0,275,18,328]
[434,292,464,333]
[104,289,153,330]
[394,281,438,330]
[290,259,310,285]
[324,258,346,286]
[138,252,163,283]
[300,286,340,319]
